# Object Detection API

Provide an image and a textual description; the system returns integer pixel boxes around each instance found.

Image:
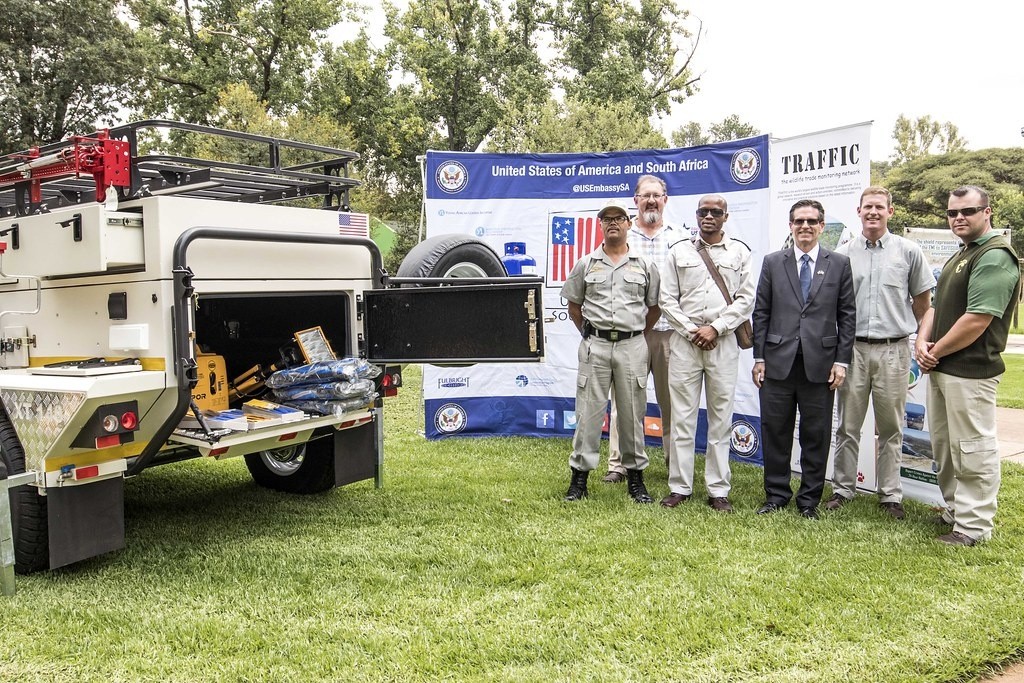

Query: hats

[597,205,630,221]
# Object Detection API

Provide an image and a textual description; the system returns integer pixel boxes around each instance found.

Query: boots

[564,466,589,502]
[627,468,654,504]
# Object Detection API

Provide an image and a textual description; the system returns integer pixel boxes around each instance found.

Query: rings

[698,341,703,345]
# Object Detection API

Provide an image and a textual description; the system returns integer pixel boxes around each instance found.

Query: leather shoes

[757,502,779,514]
[799,506,819,520]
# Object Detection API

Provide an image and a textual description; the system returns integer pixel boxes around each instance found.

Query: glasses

[636,193,665,201]
[946,205,988,217]
[696,208,728,217]
[601,216,629,224]
[793,218,821,226]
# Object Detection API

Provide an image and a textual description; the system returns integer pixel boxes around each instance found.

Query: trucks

[0,118,547,578]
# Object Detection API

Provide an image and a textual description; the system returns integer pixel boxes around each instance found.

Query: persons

[657,192,756,511]
[603,173,694,483]
[821,188,937,520]
[914,185,1022,546]
[752,199,856,517]
[557,200,661,503]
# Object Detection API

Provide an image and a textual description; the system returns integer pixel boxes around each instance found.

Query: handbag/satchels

[734,319,754,349]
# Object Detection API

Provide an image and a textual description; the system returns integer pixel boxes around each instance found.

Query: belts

[589,328,643,342]
[855,335,906,344]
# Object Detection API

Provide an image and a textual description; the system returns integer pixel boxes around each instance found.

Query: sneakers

[708,495,734,512]
[602,471,625,484]
[826,491,847,510]
[932,516,947,526]
[660,490,688,507]
[936,530,976,546]
[881,501,905,519]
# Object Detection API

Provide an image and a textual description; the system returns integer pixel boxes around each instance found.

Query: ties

[800,255,812,306]
